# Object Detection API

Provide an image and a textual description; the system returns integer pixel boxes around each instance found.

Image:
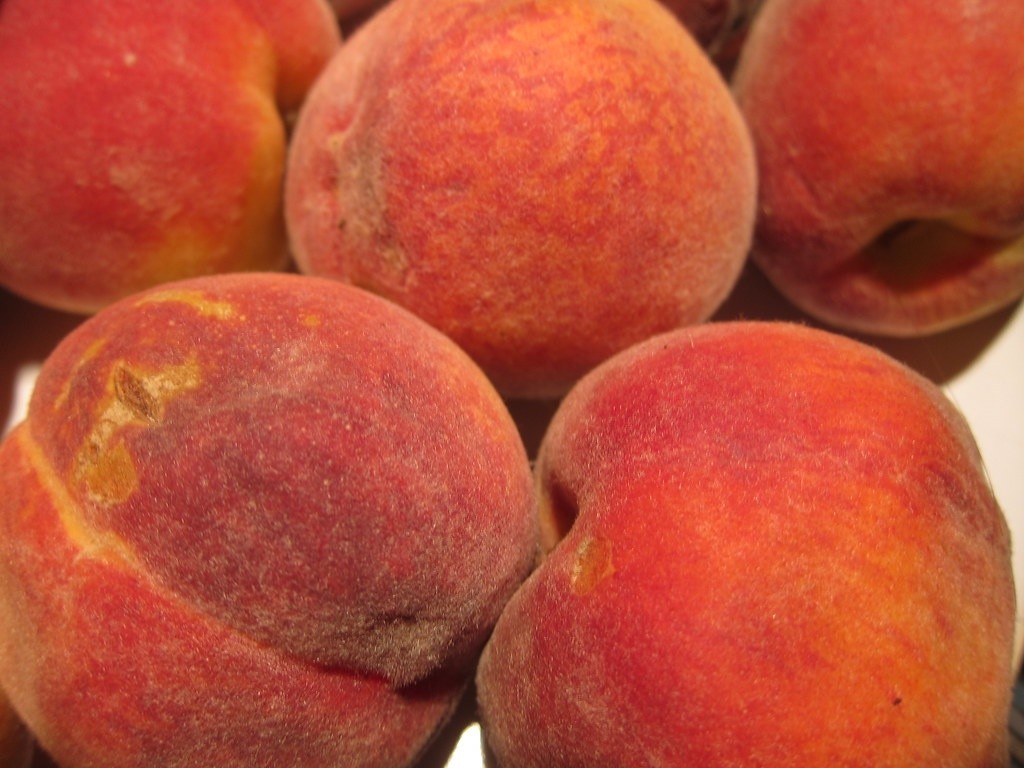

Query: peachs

[0,0,1024,768]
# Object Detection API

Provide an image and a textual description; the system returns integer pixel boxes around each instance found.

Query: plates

[0,282,1024,768]
